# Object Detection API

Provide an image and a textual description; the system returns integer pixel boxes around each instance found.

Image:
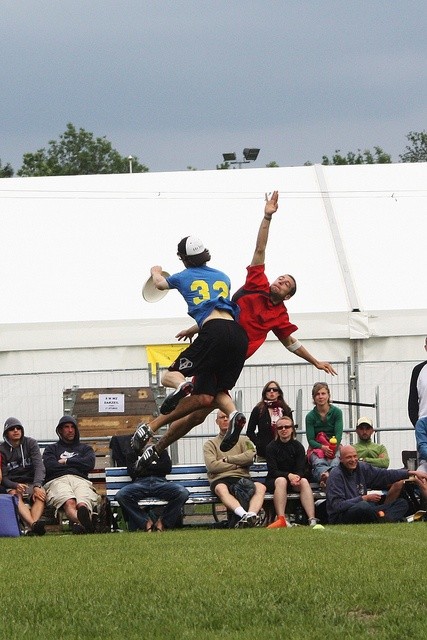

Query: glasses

[265,387,280,392]
[275,425,293,430]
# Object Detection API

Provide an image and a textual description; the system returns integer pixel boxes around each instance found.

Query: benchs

[104,461,328,531]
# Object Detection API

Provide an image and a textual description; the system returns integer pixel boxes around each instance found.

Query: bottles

[329,436,338,459]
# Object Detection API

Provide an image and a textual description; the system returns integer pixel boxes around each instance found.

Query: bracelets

[222,458,225,462]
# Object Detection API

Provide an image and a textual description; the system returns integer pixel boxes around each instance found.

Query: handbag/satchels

[0,492,21,538]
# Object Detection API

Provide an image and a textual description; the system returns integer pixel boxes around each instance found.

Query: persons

[114,435,190,532]
[128,191,338,476]
[0,417,47,537]
[325,444,426,524]
[407,336,426,460]
[149,234,246,453]
[350,417,389,468]
[304,381,342,487]
[265,416,320,528]
[202,410,267,529]
[245,380,296,459]
[41,415,95,533]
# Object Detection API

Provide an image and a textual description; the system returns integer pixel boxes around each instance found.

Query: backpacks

[229,478,256,512]
[92,494,120,534]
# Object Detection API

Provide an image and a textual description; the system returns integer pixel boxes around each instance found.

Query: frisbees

[142,271,171,303]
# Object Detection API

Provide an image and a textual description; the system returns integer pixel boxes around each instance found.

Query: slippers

[153,520,164,533]
[145,519,154,533]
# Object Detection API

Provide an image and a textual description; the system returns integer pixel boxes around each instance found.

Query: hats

[178,236,206,261]
[356,416,373,428]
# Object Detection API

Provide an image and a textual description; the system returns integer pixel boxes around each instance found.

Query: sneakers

[135,447,158,473]
[71,522,86,534]
[25,528,37,536]
[77,506,95,531]
[32,520,46,535]
[159,381,193,415]
[130,424,149,451]
[308,517,320,528]
[245,513,260,526]
[220,411,246,452]
[237,520,248,528]
